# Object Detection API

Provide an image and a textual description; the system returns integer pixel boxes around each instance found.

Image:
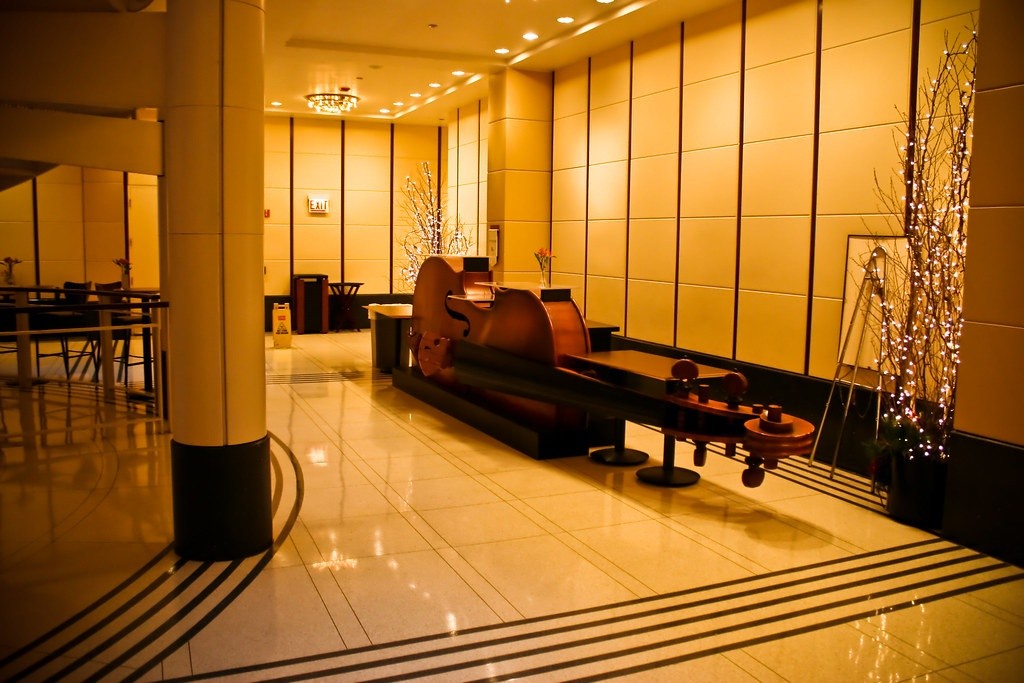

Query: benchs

[474,282,578,301]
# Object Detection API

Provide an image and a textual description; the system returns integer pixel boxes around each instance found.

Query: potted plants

[862,408,952,523]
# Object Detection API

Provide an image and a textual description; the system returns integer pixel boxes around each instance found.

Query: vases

[120,274,129,289]
[540,279,546,288]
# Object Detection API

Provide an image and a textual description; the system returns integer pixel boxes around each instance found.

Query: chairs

[36,281,127,382]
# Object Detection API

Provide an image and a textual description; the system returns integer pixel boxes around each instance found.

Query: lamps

[304,93,359,114]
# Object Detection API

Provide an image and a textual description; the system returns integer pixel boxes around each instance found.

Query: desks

[361,304,413,373]
[448,293,495,301]
[565,349,748,487]
[328,283,365,333]
[585,320,620,353]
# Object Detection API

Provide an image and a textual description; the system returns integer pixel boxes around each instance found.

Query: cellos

[406,255,817,488]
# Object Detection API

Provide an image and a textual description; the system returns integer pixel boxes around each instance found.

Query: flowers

[0,257,22,285]
[534,247,557,288]
[113,258,131,274]
